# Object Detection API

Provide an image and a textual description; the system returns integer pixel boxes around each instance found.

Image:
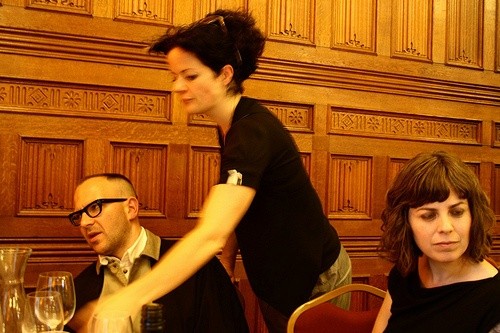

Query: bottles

[0,247,37,333]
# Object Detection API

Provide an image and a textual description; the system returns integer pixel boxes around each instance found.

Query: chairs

[287,284,387,333]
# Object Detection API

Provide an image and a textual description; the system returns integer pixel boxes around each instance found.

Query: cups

[22,291,64,333]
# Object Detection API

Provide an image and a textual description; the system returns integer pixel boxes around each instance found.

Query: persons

[87,10,352,333]
[372,152,500,333]
[63,173,250,333]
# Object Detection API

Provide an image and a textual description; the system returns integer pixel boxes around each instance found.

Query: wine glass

[36,271,76,333]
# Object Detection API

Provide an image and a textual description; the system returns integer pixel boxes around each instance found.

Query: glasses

[189,16,241,61]
[67,198,126,226]
